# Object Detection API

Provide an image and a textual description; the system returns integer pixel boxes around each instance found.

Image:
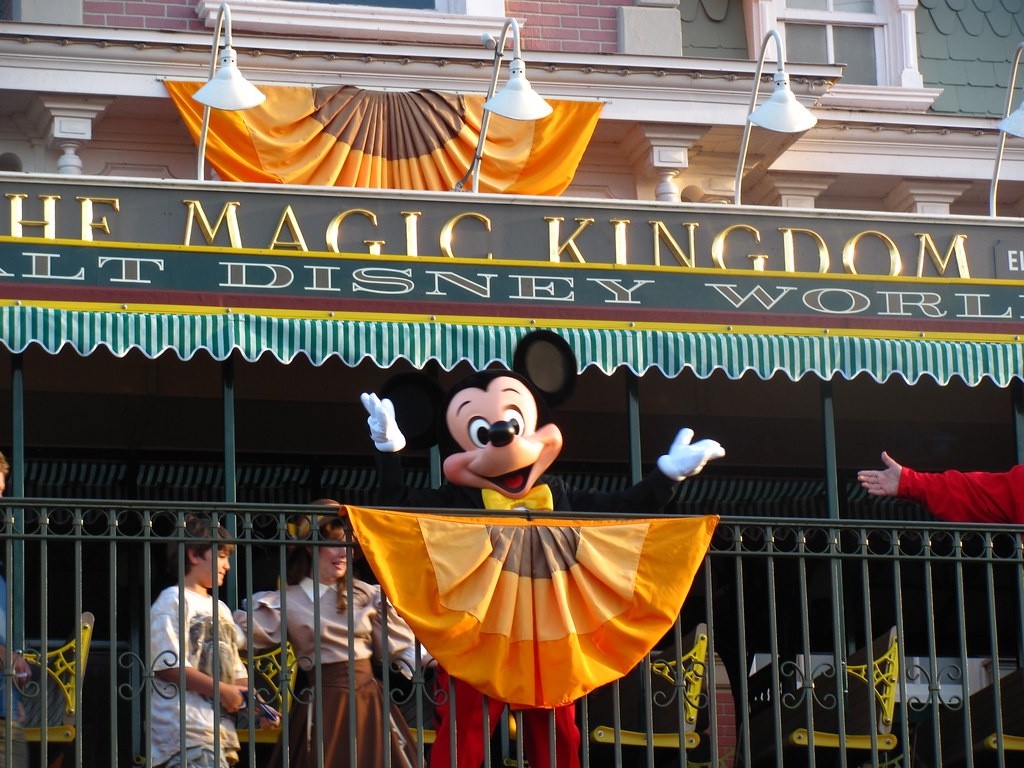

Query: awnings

[0,232,1024,389]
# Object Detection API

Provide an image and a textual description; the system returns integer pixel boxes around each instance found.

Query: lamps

[190,3,266,180]
[990,42,1024,217]
[471,17,553,194]
[734,30,818,206]
[482,33,497,50]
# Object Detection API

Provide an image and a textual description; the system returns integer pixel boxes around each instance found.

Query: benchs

[234,640,298,743]
[589,622,708,749]
[15,611,95,742]
[745,625,900,768]
[912,665,1024,768]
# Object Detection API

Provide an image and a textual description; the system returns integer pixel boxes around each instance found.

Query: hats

[286,498,361,552]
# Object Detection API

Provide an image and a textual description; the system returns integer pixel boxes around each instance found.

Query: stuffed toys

[359,331,727,768]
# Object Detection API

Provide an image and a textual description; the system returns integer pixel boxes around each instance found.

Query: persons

[857,451,1024,546]
[0,452,33,768]
[233,498,438,768]
[150,518,283,768]
